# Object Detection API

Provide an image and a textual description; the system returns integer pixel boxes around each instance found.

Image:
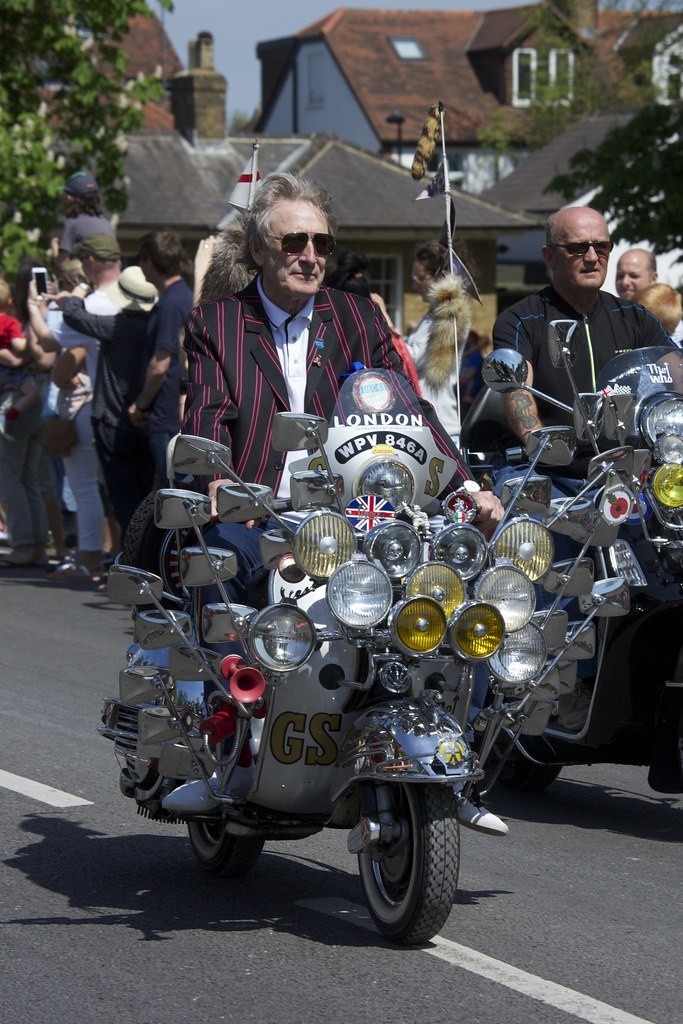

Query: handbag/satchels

[46,413,76,454]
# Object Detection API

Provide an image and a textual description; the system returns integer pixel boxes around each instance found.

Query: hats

[56,172,100,197]
[71,233,121,261]
[106,266,158,311]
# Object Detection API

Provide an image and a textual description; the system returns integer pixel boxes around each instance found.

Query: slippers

[0,560,49,569]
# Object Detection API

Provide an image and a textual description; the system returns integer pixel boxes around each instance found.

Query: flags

[228,147,262,211]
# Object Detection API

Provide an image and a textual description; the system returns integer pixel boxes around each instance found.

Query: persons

[161,170,510,837]
[0,171,195,580]
[328,206,683,729]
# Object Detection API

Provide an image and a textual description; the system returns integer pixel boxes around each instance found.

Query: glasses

[411,271,431,284]
[547,239,614,256]
[265,232,336,255]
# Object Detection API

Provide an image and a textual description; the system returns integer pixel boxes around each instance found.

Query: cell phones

[32,267,48,300]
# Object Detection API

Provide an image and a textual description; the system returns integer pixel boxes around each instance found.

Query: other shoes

[47,552,114,580]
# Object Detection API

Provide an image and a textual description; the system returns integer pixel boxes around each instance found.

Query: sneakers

[162,758,255,812]
[453,780,508,836]
[558,678,592,731]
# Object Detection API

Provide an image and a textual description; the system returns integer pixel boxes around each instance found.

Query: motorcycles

[435,316,683,809]
[106,367,649,944]
